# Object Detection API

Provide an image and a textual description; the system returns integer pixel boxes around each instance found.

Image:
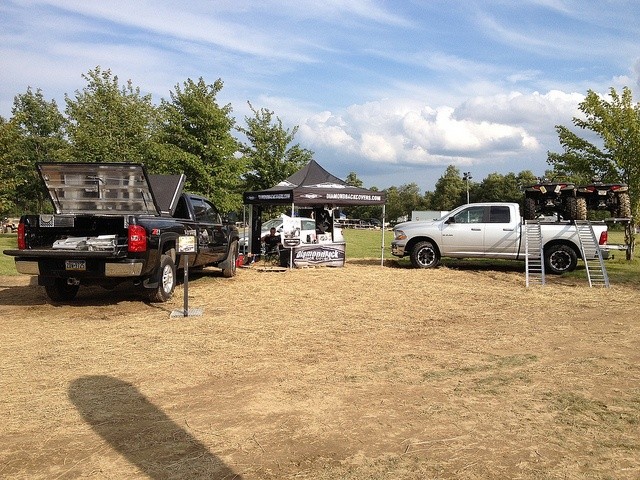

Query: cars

[237,218,343,251]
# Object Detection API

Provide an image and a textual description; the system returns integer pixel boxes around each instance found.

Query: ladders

[525,219,545,288]
[574,219,610,288]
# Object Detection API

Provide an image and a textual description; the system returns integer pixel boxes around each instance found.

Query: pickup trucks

[391,202,608,273]
[3,162,240,301]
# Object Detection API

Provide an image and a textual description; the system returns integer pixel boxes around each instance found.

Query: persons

[261,227,280,256]
